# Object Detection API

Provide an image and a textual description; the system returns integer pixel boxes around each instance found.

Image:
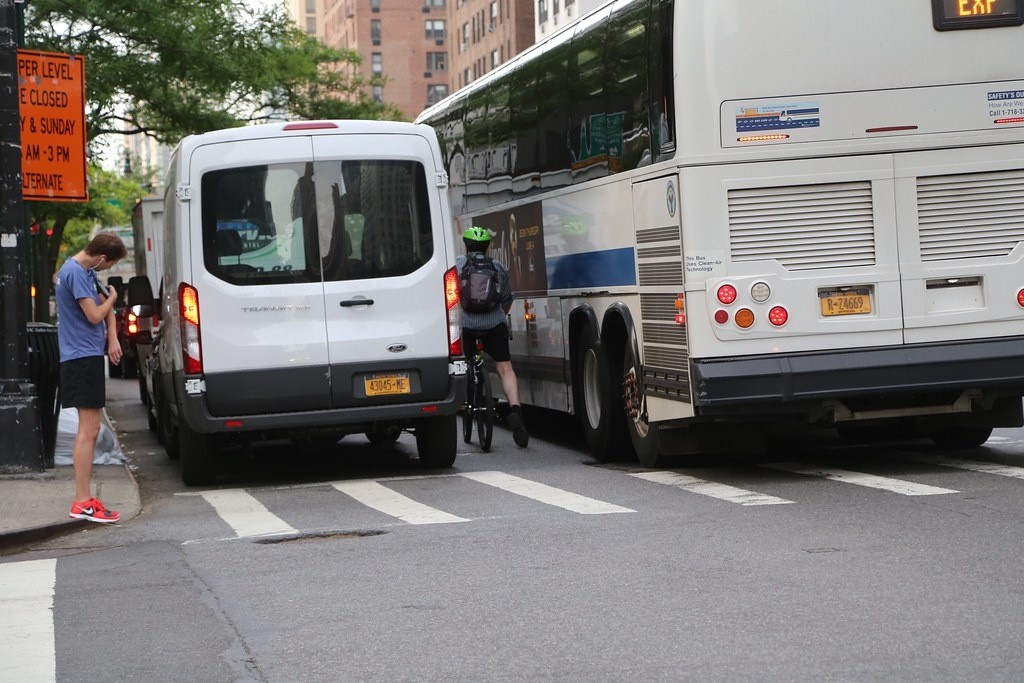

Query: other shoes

[507,413,529,448]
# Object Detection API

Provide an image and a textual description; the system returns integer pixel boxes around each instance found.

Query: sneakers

[69,497,121,523]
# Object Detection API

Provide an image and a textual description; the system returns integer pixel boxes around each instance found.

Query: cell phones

[98,282,110,296]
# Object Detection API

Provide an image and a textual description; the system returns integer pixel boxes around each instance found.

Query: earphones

[101,257,105,263]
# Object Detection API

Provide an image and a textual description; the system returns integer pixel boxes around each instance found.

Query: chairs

[215,229,258,275]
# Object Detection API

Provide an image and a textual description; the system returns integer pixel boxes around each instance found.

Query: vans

[92,120,467,472]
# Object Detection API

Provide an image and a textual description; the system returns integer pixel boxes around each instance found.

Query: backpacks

[459,253,502,313]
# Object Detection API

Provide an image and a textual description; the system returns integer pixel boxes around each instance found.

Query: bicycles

[462,336,497,451]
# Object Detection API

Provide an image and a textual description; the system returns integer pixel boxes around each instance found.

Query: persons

[456,227,529,447]
[55,233,126,524]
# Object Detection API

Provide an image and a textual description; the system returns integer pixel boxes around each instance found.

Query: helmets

[463,226,491,252]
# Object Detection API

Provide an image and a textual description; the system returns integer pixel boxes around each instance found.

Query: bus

[414,0,1024,467]
